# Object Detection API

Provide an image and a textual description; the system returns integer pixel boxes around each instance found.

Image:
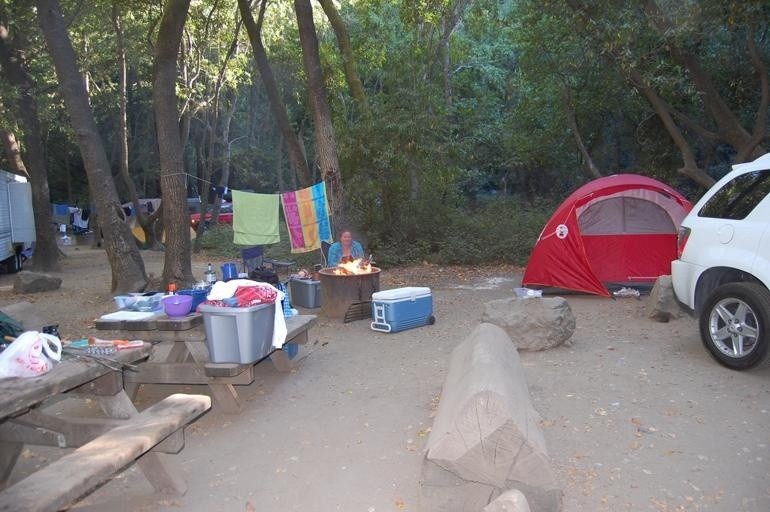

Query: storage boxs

[77,230,93,245]
[370,286,433,334]
[198,299,275,364]
[113,293,165,312]
[290,278,320,308]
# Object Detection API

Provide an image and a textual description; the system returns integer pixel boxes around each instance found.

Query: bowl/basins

[163,290,208,317]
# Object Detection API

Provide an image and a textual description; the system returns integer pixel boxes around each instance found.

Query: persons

[327,230,365,268]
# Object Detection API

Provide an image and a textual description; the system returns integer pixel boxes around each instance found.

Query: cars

[191,202,233,233]
[670,152,770,371]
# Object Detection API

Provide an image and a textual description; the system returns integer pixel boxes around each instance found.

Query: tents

[515,173,693,300]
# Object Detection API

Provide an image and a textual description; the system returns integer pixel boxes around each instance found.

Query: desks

[0,341,152,423]
[95,309,207,342]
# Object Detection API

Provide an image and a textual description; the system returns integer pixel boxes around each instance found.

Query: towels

[231,189,280,245]
[281,180,334,254]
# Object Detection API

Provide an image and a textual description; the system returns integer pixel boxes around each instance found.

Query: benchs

[205,312,316,385]
[0,393,210,512]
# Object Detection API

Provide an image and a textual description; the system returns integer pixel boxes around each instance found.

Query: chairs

[237,246,291,288]
[320,241,332,268]
